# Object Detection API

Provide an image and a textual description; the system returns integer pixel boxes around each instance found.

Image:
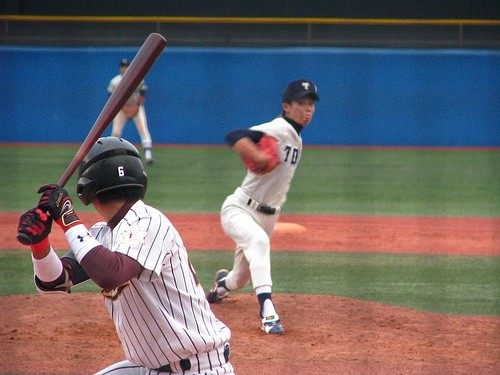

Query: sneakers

[261,314,284,334]
[206,269,229,304]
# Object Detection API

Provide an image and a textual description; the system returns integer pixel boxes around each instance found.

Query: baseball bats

[16,32,168,246]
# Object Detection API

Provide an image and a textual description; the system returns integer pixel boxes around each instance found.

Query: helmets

[76,136,147,204]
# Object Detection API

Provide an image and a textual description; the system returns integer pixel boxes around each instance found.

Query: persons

[205,80,320,334]
[16,137,235,375]
[106,57,153,166]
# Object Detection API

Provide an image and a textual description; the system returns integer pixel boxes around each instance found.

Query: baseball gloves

[240,136,280,174]
[124,104,141,120]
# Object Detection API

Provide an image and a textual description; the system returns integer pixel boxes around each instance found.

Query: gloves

[37,185,82,232]
[17,209,52,253]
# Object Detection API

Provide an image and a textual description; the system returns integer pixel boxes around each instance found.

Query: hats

[284,79,319,103]
[119,57,129,66]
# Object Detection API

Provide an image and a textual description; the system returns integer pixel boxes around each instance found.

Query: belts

[155,345,231,373]
[248,198,276,215]
